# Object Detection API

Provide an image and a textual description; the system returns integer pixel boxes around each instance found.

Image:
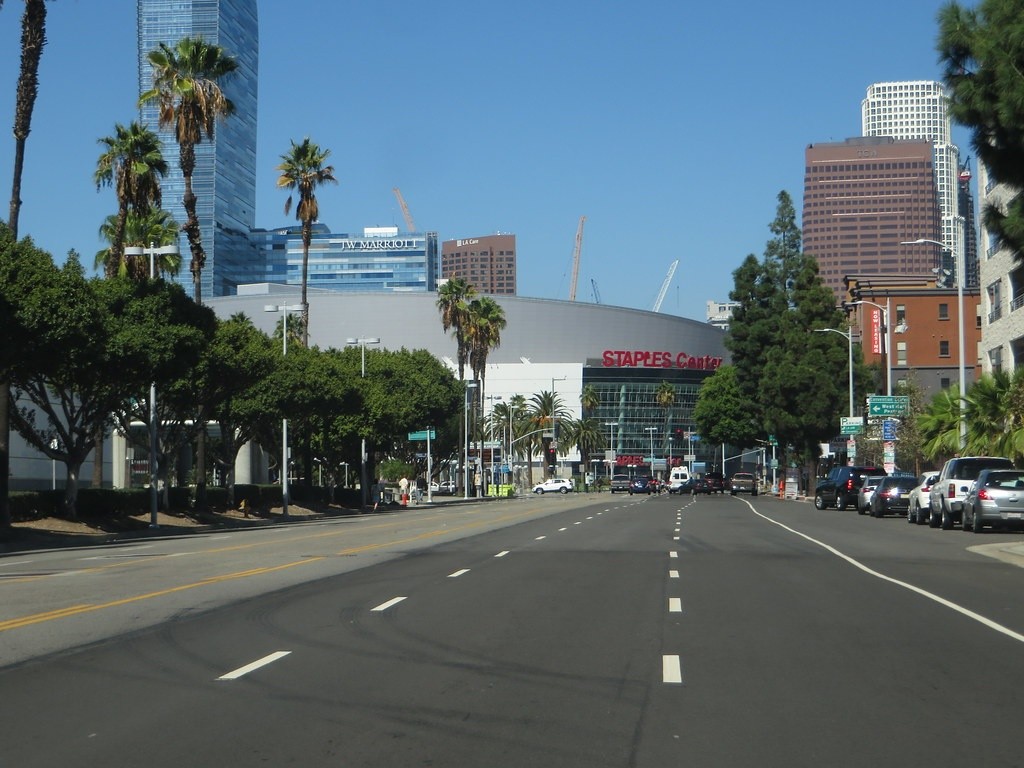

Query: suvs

[928,456,1017,530]
[814,464,888,510]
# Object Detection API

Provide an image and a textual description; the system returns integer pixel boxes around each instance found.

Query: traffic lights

[676,428,683,440]
[549,449,555,465]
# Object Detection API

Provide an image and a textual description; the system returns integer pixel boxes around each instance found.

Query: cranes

[390,186,418,231]
[557,215,587,301]
[648,260,682,314]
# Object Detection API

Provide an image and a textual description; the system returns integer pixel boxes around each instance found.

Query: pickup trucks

[610,474,632,493]
[731,472,758,496]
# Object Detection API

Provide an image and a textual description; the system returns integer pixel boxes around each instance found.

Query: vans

[668,467,691,492]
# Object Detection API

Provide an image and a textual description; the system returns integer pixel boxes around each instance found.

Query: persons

[371,479,381,511]
[399,475,409,493]
[417,475,425,501]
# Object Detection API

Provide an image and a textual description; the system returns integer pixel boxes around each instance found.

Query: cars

[532,478,574,494]
[423,480,457,495]
[629,475,667,495]
[678,473,725,496]
[856,472,942,525]
[961,467,1024,533]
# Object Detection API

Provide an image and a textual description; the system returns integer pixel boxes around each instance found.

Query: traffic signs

[869,395,910,417]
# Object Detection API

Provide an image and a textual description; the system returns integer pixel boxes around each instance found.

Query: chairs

[1015,480,1024,487]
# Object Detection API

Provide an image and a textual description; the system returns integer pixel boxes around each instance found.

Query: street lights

[124,241,180,527]
[486,394,502,498]
[345,336,381,507]
[846,297,894,477]
[551,377,565,442]
[508,405,520,471]
[263,301,303,519]
[605,421,619,479]
[814,326,854,465]
[899,238,968,456]
[644,425,658,477]
